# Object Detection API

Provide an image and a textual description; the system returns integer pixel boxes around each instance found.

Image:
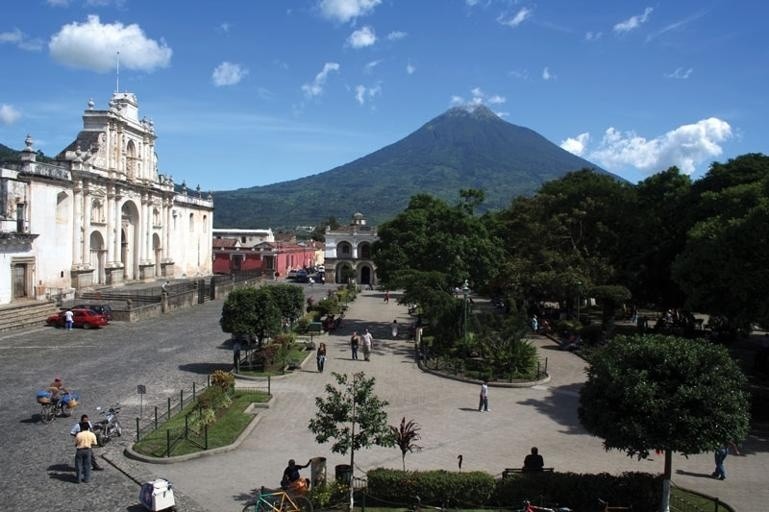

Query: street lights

[461,278,470,374]
[575,278,582,338]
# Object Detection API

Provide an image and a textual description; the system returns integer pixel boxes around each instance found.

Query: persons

[477,379,492,412]
[362,328,374,362]
[232,337,242,367]
[520,447,545,472]
[531,314,539,339]
[350,330,360,360]
[279,457,312,490]
[49,376,70,410]
[708,440,740,480]
[286,471,308,496]
[384,289,390,304]
[69,413,103,471]
[275,271,279,281]
[63,307,74,333]
[390,319,400,341]
[316,342,327,374]
[323,319,336,336]
[72,423,98,484]
[407,321,417,343]
[414,323,423,344]
[161,280,170,296]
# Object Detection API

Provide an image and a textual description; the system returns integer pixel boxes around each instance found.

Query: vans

[65,303,113,322]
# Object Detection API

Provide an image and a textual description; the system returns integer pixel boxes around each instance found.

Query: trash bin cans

[335,464,353,488]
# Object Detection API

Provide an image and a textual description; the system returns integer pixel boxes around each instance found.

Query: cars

[47,309,108,330]
[287,264,325,284]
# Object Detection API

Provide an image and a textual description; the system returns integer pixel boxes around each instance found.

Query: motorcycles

[94,401,123,446]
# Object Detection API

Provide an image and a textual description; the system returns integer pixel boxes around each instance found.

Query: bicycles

[513,496,573,512]
[593,496,636,512]
[403,493,452,511]
[40,391,74,424]
[241,480,314,511]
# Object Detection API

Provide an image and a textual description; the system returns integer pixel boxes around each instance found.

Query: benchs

[502,468,554,480]
